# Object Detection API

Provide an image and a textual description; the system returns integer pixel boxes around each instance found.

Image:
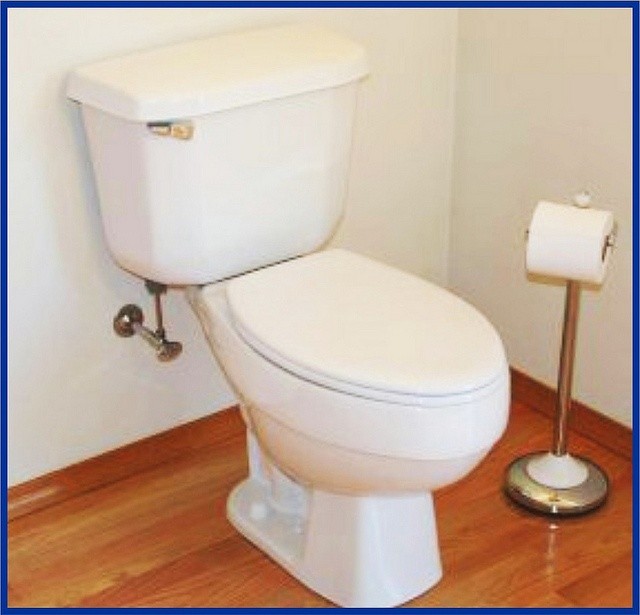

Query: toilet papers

[524,196,615,288]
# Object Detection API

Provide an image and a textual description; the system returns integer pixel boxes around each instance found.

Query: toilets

[67,27,514,608]
